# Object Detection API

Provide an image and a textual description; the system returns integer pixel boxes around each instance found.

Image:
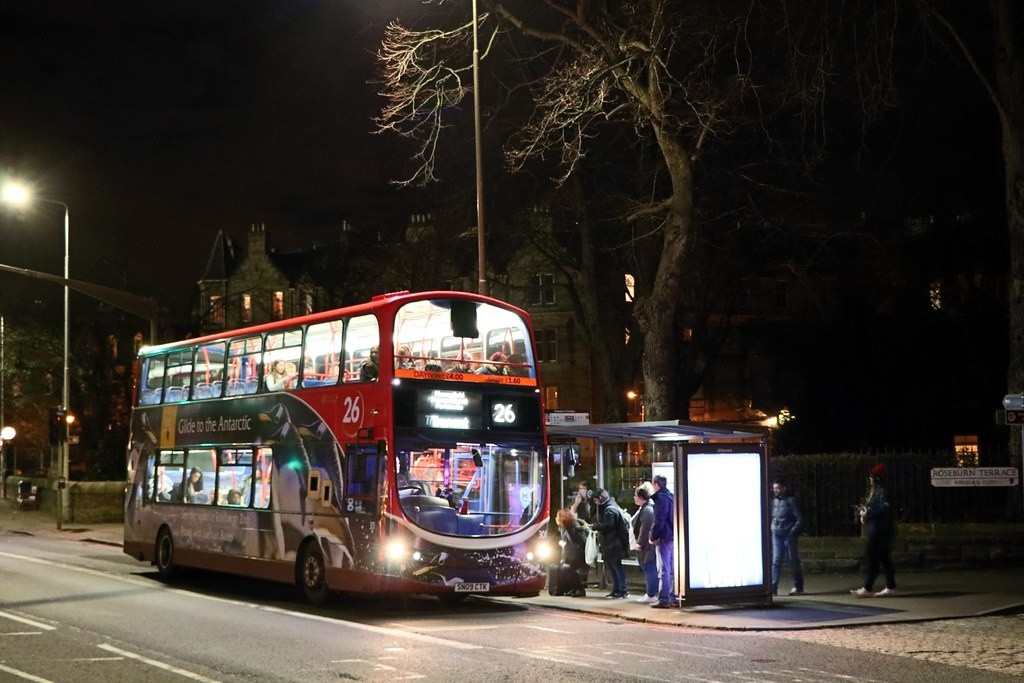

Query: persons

[372,456,408,489]
[148,466,263,507]
[850,464,896,595]
[555,475,679,608]
[768,479,804,594]
[263,343,529,391]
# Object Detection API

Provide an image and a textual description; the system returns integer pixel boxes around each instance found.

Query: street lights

[1,180,74,525]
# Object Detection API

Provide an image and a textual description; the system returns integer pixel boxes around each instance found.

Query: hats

[870,463,886,478]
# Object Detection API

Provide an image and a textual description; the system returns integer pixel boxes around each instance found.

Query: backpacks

[606,504,632,533]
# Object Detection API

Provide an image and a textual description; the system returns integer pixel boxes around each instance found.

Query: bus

[118,291,552,615]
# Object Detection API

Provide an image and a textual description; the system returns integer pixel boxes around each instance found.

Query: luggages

[549,563,570,596]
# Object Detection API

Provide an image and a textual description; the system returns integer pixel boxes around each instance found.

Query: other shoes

[650,601,678,608]
[872,588,902,597]
[571,590,585,596]
[637,593,658,602]
[564,589,573,596]
[788,587,804,596]
[606,591,630,600]
[850,587,873,597]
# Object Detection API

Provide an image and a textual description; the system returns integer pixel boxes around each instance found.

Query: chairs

[15,484,38,512]
[151,369,349,407]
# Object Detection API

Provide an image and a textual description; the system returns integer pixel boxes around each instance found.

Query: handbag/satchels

[585,529,599,568]
